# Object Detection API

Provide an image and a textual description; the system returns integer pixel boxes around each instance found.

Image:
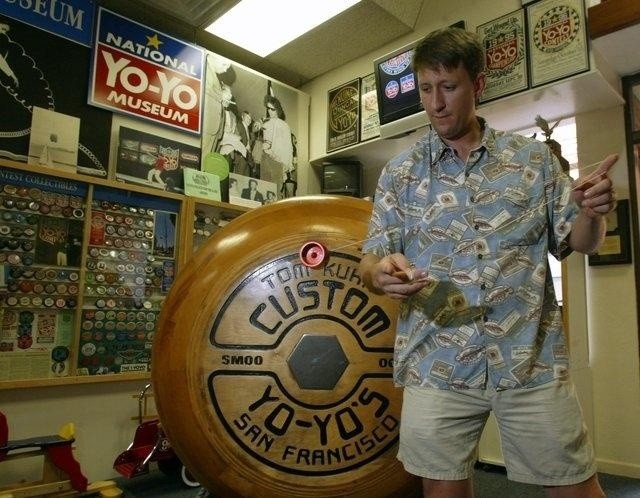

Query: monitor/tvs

[368,17,469,142]
[320,160,364,198]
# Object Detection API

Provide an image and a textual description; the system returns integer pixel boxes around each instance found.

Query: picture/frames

[152,210,178,261]
[522,0,590,88]
[360,73,380,142]
[326,77,361,153]
[475,8,529,105]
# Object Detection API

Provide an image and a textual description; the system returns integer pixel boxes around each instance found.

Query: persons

[146,151,165,185]
[56,239,70,267]
[262,191,276,206]
[234,111,252,177]
[229,178,237,189]
[359,28,620,498]
[218,81,248,176]
[255,94,295,197]
[241,179,263,202]
[201,53,232,172]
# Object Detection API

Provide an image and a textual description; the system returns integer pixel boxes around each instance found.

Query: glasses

[267,107,278,111]
